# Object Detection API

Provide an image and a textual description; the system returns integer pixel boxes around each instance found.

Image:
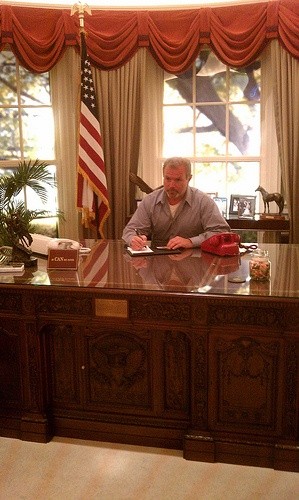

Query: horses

[254,186,285,215]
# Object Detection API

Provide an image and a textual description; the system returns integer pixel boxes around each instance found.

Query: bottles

[248,249,271,281]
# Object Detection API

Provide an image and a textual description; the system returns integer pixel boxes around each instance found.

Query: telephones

[19,233,82,256]
[201,233,241,256]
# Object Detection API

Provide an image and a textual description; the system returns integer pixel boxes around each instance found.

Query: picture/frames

[229,194,256,217]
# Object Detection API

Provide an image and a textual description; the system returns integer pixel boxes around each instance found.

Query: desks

[0,239,299,473]
[224,214,291,230]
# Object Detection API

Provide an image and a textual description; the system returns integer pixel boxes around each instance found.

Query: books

[126,244,182,257]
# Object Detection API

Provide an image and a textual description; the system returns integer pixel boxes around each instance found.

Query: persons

[132,249,217,293]
[122,157,232,250]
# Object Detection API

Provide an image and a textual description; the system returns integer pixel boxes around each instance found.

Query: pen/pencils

[135,229,147,249]
[155,246,167,249]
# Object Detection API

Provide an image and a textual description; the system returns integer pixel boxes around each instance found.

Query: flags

[80,240,110,288]
[75,29,112,239]
